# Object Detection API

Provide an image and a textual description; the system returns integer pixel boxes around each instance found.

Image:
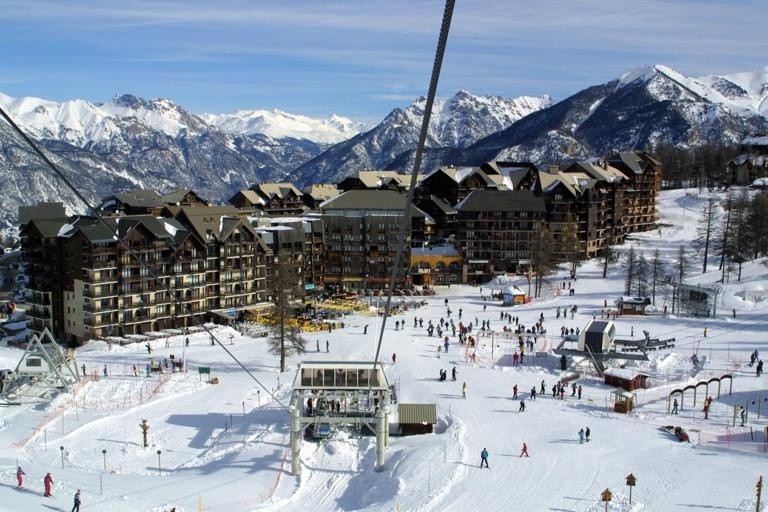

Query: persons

[103,364,108,376]
[423,284,428,289]
[341,322,345,328]
[664,306,667,313]
[186,337,189,346]
[592,309,619,320]
[520,443,529,457]
[43,473,54,497]
[132,364,138,376]
[364,324,369,333]
[326,341,329,352]
[512,380,583,411]
[393,352,396,362]
[316,340,320,351]
[480,448,489,468]
[81,363,86,375]
[704,396,712,418]
[750,349,763,376]
[440,366,456,381]
[704,328,707,337]
[211,335,216,346]
[672,399,678,414]
[395,296,580,369]
[579,427,590,444]
[740,406,746,426]
[733,309,736,318]
[229,334,234,345]
[631,326,634,336]
[563,273,576,296]
[462,382,467,398]
[71,489,81,511]
[332,322,337,330]
[17,467,26,489]
[328,322,332,332]
[166,336,169,348]
[147,342,152,353]
